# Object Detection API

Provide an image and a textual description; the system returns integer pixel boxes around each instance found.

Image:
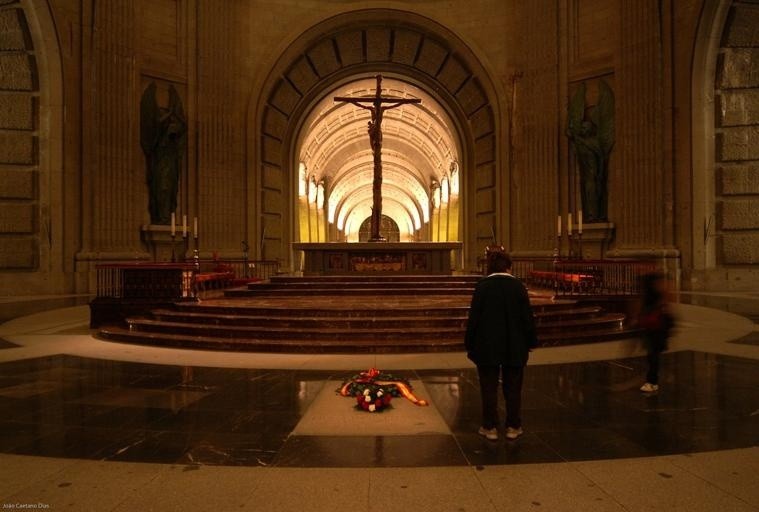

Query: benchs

[526,270,593,293]
[197,270,228,291]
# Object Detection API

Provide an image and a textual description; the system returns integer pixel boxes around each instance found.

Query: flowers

[335,368,413,411]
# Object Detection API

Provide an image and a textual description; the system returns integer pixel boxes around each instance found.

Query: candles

[567,213,572,235]
[557,215,561,236]
[193,216,198,238]
[170,212,176,236]
[578,211,582,233]
[182,215,188,238]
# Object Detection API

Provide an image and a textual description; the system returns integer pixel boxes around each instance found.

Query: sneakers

[504,426,524,439]
[639,382,659,392]
[478,425,499,440]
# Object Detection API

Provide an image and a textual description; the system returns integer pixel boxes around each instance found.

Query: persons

[464,250,538,440]
[630,273,676,392]
